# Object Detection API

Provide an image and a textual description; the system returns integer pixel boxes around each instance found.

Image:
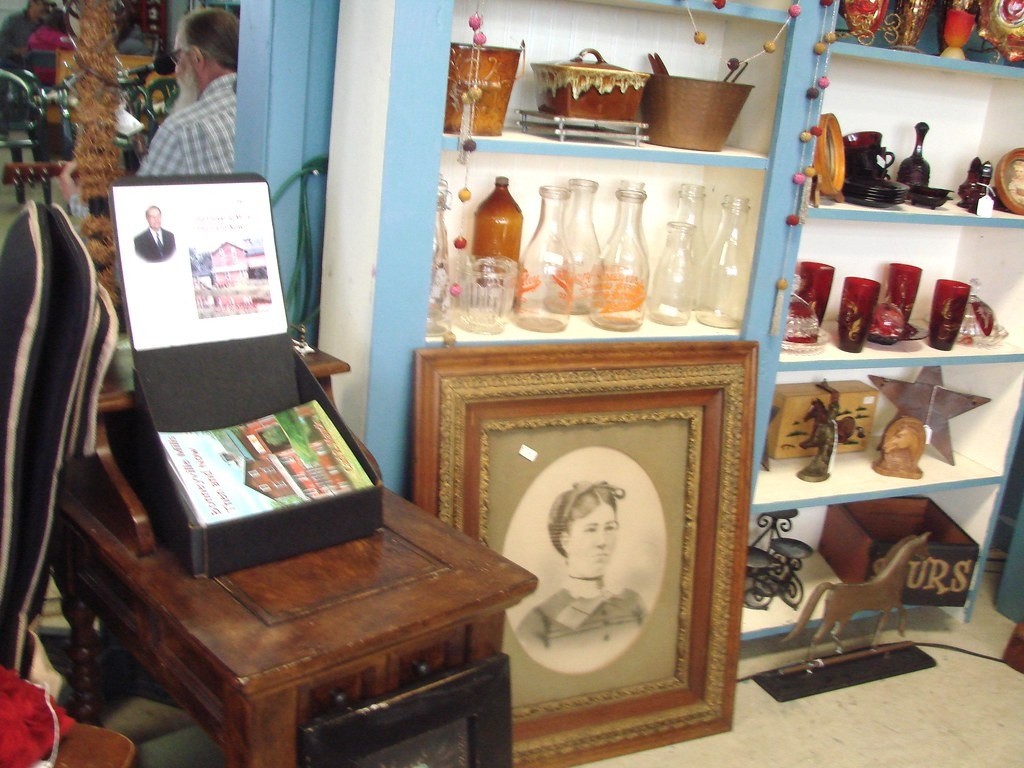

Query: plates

[844,175,910,205]
[868,323,917,347]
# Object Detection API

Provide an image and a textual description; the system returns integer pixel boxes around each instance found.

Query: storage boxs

[766,377,879,458]
[819,495,981,607]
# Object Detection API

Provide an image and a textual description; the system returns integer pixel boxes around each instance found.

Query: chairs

[0,43,181,200]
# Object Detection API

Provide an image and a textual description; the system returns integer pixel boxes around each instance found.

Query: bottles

[696,198,750,333]
[590,189,649,333]
[429,179,457,334]
[473,177,522,309]
[511,187,572,333]
[653,221,699,326]
[546,181,605,316]
[673,189,708,266]
[896,123,931,186]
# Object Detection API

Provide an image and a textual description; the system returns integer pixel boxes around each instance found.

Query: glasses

[168,47,187,65]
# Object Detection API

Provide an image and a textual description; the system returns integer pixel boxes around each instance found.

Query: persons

[0,0,75,161]
[134,206,176,263]
[1008,160,1024,207]
[58,8,240,224]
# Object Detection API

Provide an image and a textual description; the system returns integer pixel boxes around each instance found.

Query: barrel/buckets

[643,60,752,152]
[443,44,527,135]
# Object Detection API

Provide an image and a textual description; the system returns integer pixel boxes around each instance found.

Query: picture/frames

[415,339,760,768]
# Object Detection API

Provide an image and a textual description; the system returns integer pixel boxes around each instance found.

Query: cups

[844,119,898,167]
[791,263,836,341]
[890,263,924,320]
[931,281,970,349]
[836,277,883,351]
[458,258,518,331]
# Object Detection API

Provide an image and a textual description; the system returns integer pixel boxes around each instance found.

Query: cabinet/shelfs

[57,344,541,768]
[313,0,1024,656]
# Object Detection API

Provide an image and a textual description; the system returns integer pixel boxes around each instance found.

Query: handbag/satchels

[0,199,119,682]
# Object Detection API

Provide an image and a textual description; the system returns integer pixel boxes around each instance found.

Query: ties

[157,233,163,252]
